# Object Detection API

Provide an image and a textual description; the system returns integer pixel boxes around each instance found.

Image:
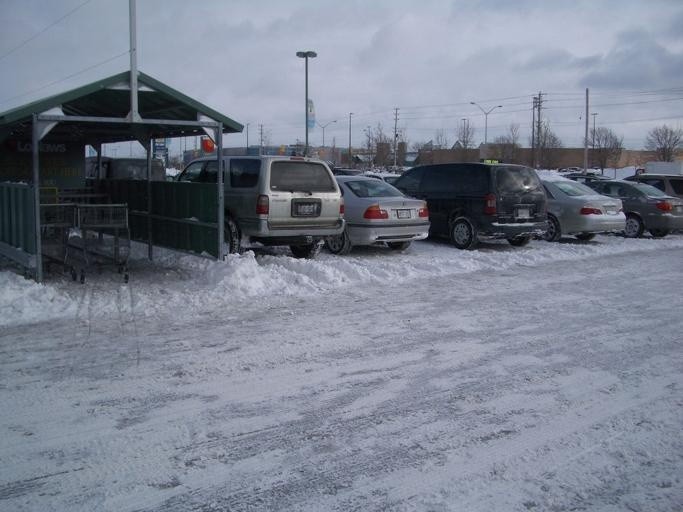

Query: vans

[164,155,346,260]
[391,162,549,250]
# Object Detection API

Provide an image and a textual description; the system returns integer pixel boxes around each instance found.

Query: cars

[582,179,682,238]
[623,173,682,234]
[322,175,431,256]
[538,176,626,242]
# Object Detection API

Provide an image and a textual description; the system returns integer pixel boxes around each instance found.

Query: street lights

[470,101,502,144]
[308,119,336,151]
